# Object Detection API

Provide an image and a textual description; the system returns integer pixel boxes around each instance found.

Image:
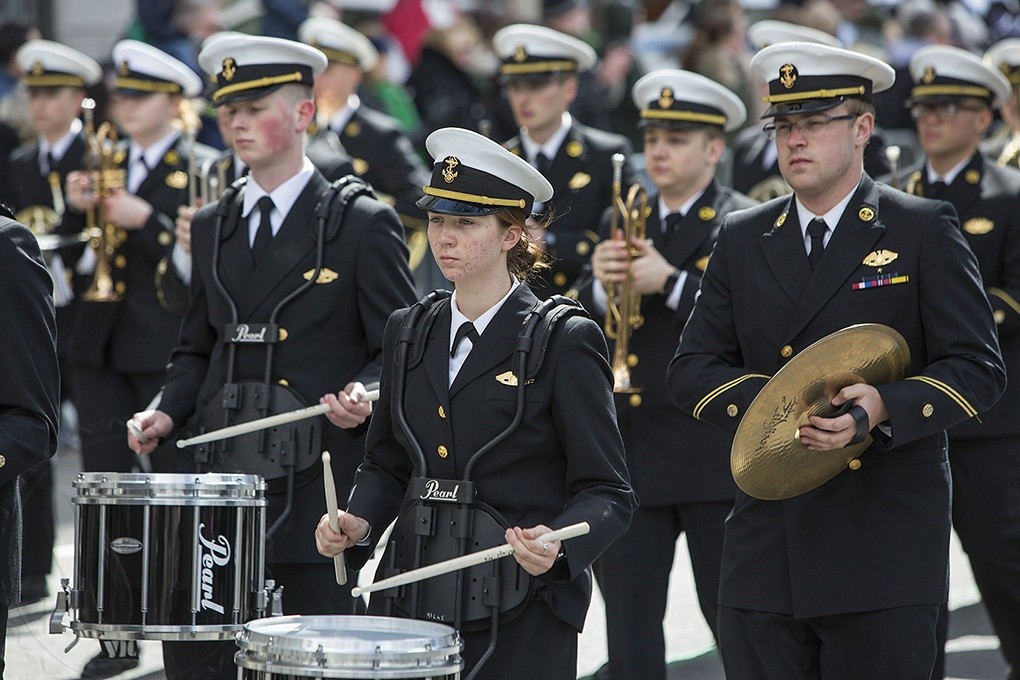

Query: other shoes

[19,575,47,598]
[83,649,138,678]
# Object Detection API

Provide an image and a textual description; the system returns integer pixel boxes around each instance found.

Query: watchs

[663,274,679,295]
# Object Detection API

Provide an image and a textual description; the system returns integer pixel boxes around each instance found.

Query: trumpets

[79,95,128,303]
[156,108,237,316]
[604,153,646,394]
[13,169,67,239]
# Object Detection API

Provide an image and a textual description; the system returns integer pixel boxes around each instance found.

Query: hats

[908,49,1010,111]
[197,36,328,108]
[15,39,101,87]
[981,39,1020,86]
[415,128,554,223]
[298,17,376,74]
[632,70,746,133]
[752,42,896,118]
[750,21,839,52]
[492,24,598,83]
[111,38,201,99]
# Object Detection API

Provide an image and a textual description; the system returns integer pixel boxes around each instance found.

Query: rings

[543,543,548,556]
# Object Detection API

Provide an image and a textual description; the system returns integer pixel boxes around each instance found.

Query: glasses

[911,101,979,119]
[763,114,857,138]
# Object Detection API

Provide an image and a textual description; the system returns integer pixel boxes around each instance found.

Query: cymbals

[730,324,911,501]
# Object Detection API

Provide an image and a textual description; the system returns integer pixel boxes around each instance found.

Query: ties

[252,195,280,267]
[807,220,826,270]
[536,152,547,176]
[661,214,682,246]
[930,181,946,200]
[46,152,56,172]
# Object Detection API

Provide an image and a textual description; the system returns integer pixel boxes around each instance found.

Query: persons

[567,69,760,680]
[0,0,1020,142]
[729,17,892,205]
[297,20,426,232]
[126,37,416,680]
[492,23,634,287]
[49,41,203,680]
[0,207,63,680]
[316,127,636,680]
[668,45,1008,680]
[159,98,356,314]
[879,44,1020,680]
[7,40,104,611]
[981,39,1020,169]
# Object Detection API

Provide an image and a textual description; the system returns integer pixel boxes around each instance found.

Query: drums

[71,471,269,641]
[233,614,464,679]
